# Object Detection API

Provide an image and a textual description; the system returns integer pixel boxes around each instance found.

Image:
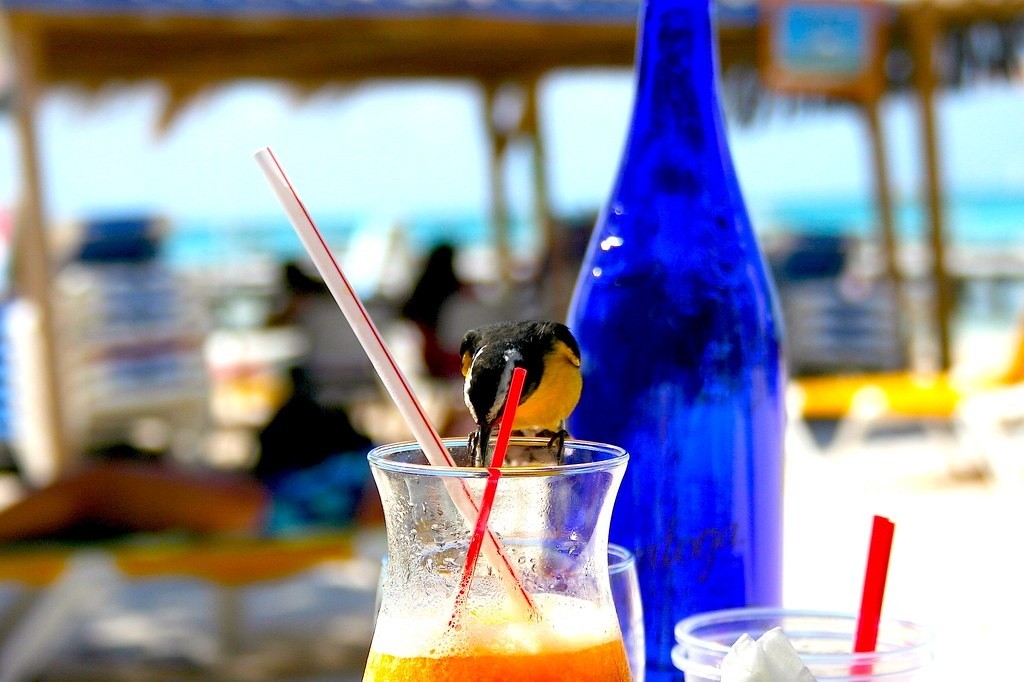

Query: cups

[669,602,925,682]
[355,432,647,682]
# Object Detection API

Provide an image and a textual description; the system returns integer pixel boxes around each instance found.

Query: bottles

[544,0,793,682]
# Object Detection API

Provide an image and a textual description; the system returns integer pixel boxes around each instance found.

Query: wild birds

[457,319,584,463]
